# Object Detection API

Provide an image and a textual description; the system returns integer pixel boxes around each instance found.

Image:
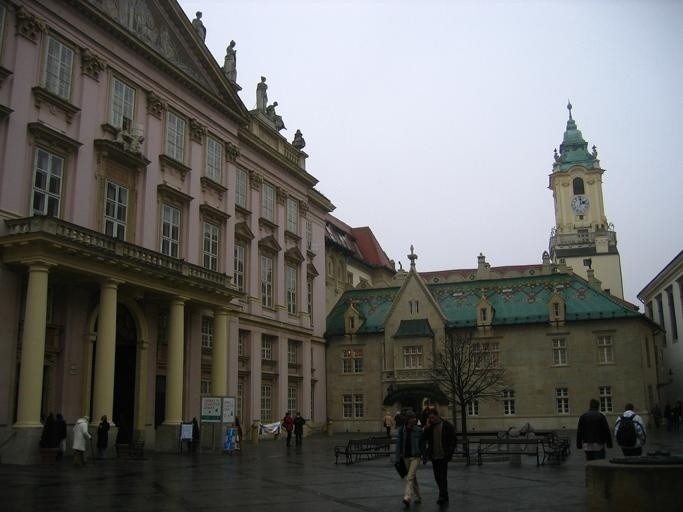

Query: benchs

[477,438,545,467]
[335,436,389,465]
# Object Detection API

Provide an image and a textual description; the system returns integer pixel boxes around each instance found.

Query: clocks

[570,194,589,216]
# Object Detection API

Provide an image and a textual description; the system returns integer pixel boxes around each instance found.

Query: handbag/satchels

[394,457,407,479]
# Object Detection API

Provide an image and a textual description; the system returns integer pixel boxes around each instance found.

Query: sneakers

[402,498,410,508]
[413,496,420,504]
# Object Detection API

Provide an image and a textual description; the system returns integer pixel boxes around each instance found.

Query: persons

[116,130,129,153]
[650,399,683,433]
[192,12,206,42]
[190,417,198,440]
[257,77,268,114]
[283,412,305,448]
[267,102,286,132]
[224,40,236,83]
[614,403,647,457]
[55,413,66,459]
[129,135,145,161]
[70,415,93,465]
[96,415,111,460]
[383,405,458,507]
[576,398,613,460]
[292,130,305,151]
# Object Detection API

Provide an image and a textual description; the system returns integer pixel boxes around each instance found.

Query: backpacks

[615,414,635,447]
